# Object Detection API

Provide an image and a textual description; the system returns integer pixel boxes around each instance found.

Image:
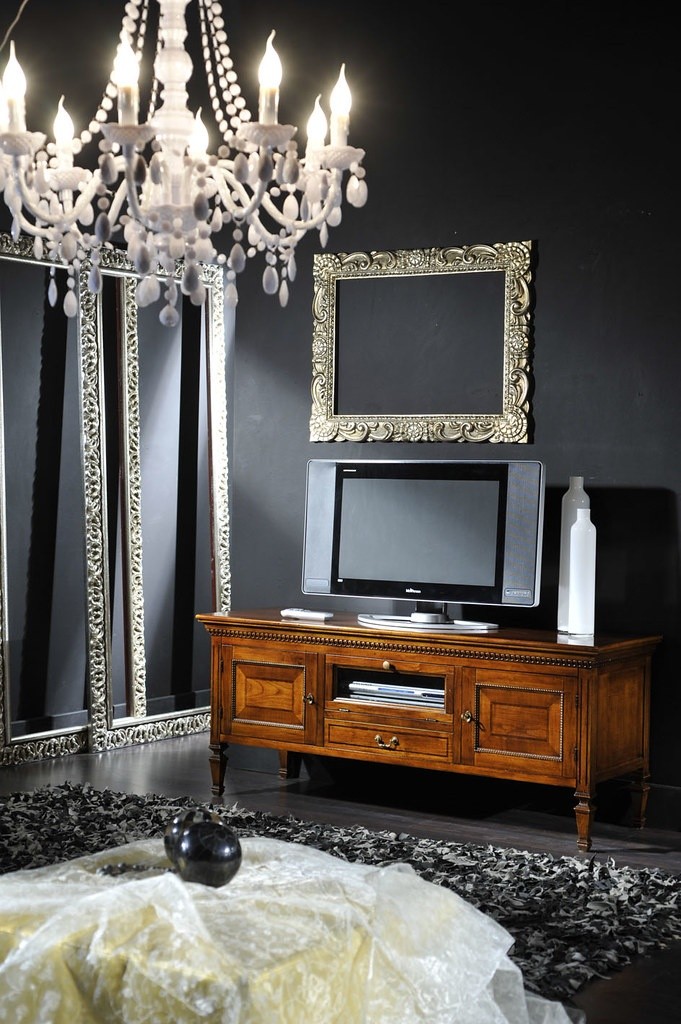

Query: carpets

[0,779,681,1006]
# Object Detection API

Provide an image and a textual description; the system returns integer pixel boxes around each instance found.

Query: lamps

[0,0,368,326]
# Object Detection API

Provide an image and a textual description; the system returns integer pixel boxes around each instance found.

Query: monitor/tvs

[301,459,546,633]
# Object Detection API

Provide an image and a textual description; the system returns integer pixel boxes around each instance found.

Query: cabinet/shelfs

[195,609,660,852]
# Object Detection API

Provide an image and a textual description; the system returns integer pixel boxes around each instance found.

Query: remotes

[281,608,334,621]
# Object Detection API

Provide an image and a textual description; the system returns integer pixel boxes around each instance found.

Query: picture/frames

[310,240,530,443]
